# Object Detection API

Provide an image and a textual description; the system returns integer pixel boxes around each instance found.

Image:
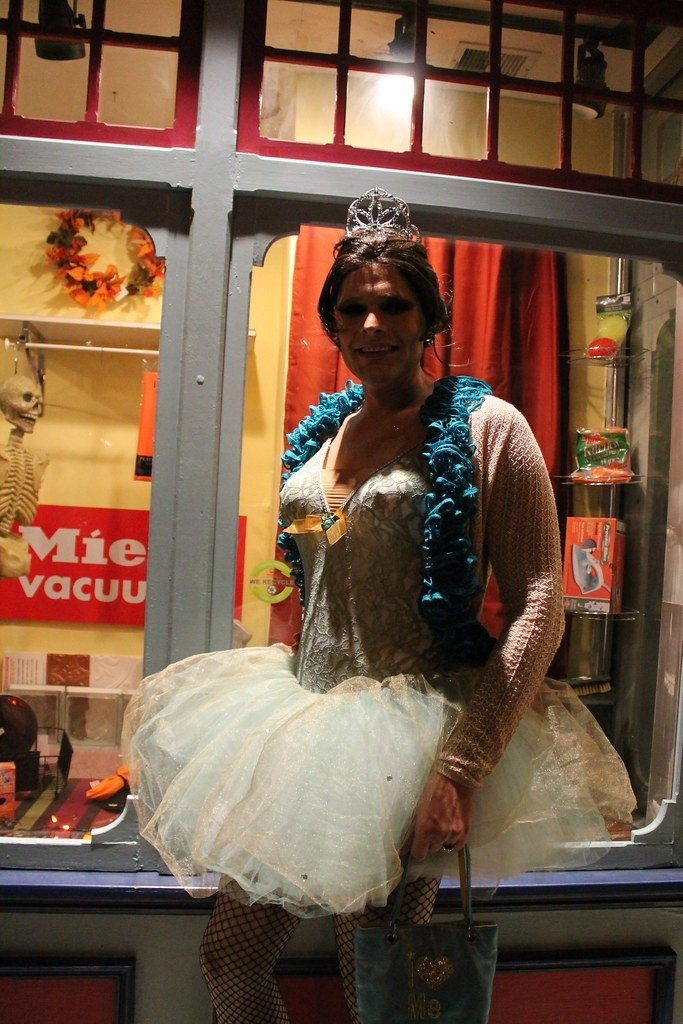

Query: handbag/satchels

[354,842,499,1024]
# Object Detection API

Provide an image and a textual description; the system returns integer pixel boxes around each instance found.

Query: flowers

[45,206,164,311]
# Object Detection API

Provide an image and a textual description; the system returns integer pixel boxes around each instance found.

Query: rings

[441,846,455,854]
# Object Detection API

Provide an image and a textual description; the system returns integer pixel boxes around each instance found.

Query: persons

[120,192,636,1024]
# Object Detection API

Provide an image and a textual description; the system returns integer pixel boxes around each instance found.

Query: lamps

[387,2,415,63]
[36,0,89,61]
[571,35,609,120]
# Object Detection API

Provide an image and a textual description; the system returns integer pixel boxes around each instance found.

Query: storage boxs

[563,516,627,613]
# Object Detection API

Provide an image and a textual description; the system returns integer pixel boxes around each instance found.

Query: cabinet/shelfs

[553,349,654,622]
